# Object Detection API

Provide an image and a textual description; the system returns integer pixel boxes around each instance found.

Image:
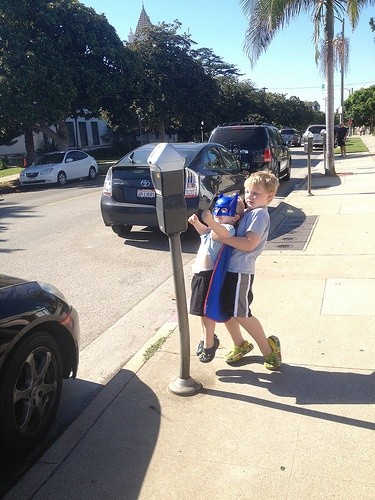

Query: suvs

[208,122,292,187]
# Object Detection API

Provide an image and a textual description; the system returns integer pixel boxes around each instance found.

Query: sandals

[262,335,282,370]
[223,340,254,363]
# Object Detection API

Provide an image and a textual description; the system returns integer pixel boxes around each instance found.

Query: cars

[304,124,327,147]
[0,275,83,461]
[19,149,98,187]
[101,142,251,239]
[278,128,302,147]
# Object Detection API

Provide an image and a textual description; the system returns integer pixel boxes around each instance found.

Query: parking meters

[303,131,314,196]
[149,147,205,396]
[320,129,328,169]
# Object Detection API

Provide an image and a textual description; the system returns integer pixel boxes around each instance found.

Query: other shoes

[198,336,220,363]
[196,333,217,356]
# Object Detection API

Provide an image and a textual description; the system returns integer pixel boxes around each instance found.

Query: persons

[212,168,282,370]
[334,122,349,158]
[188,192,244,363]
